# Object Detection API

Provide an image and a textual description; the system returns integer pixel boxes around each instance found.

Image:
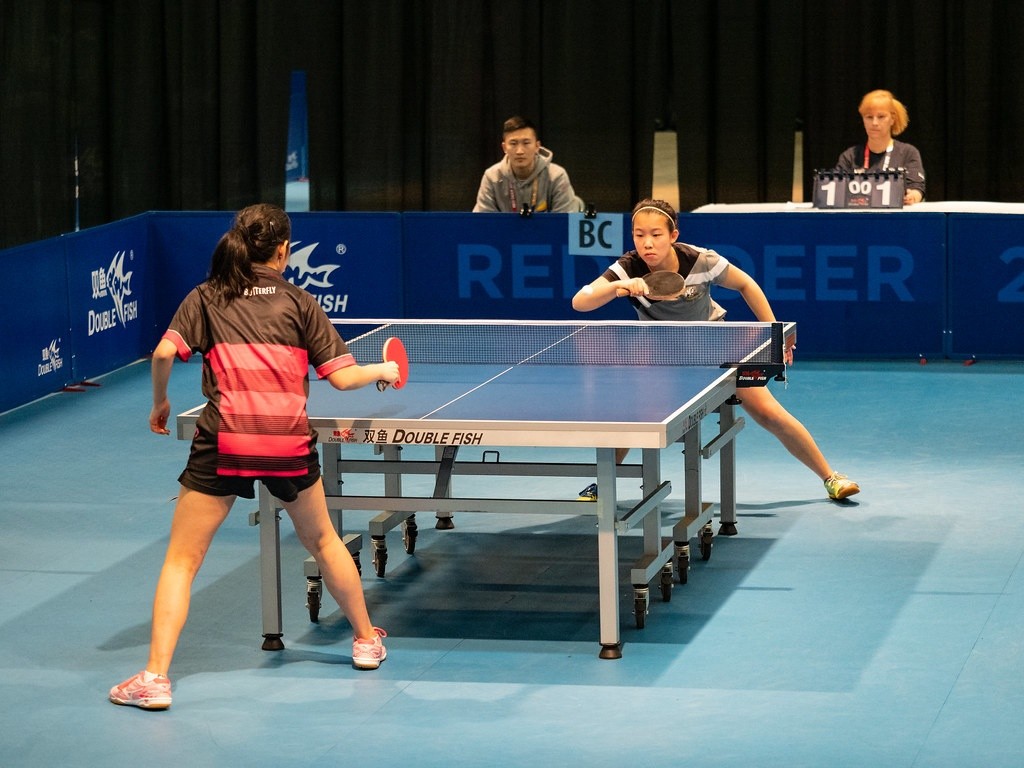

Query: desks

[689,200,1024,214]
[173,319,797,662]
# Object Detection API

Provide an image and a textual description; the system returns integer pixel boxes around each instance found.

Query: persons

[472,115,578,212]
[108,204,401,712]
[836,90,927,205]
[571,199,860,503]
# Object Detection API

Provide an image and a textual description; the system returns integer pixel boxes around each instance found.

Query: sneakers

[822,470,862,505]
[109,673,173,709]
[351,626,386,670]
[574,482,599,503]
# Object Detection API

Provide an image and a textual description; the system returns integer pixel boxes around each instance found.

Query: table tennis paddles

[376,335,410,393]
[616,270,685,300]
